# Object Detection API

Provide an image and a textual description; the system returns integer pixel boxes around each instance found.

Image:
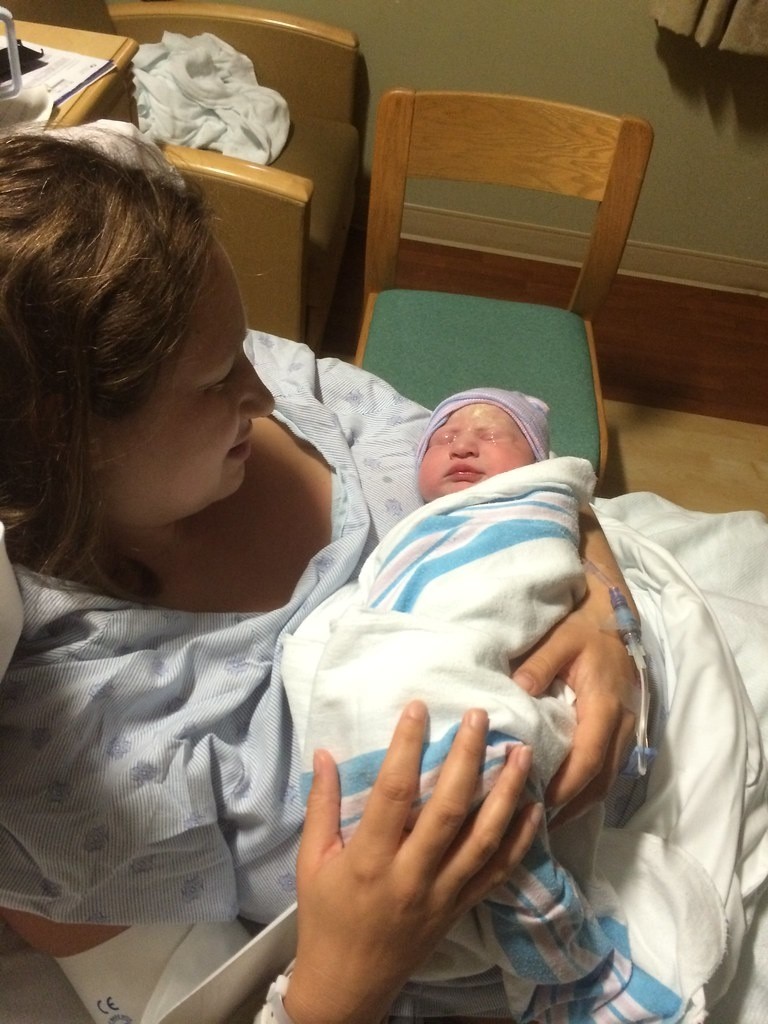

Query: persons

[0,124,768,1023]
[304,385,613,985]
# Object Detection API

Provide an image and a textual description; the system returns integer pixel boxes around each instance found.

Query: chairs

[353,87,654,497]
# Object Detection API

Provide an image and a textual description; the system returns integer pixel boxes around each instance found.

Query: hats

[416,387,551,463]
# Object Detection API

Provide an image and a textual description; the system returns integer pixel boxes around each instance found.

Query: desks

[0,18,143,130]
